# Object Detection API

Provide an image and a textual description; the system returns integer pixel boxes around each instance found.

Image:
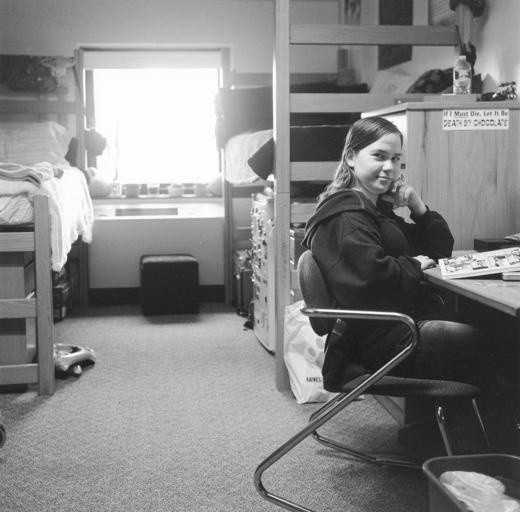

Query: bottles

[88,169,208,199]
[453,55,471,94]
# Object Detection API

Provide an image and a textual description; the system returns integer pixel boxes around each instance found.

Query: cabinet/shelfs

[357,93,518,259]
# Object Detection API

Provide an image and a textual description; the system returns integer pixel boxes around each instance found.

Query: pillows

[1,122,72,172]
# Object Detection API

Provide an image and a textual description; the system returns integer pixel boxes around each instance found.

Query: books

[437,245,519,283]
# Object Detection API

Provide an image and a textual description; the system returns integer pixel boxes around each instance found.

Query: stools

[140,254,200,316]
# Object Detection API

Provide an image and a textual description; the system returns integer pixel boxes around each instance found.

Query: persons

[301,115,489,382]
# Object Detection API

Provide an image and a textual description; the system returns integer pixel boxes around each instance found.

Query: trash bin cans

[422,454,520,512]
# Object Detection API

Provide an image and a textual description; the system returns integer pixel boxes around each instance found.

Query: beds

[218,1,472,350]
[0,52,97,400]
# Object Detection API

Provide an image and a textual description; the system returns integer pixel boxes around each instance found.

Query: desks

[415,245,520,335]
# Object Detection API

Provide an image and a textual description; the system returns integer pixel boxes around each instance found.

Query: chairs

[250,246,520,512]
